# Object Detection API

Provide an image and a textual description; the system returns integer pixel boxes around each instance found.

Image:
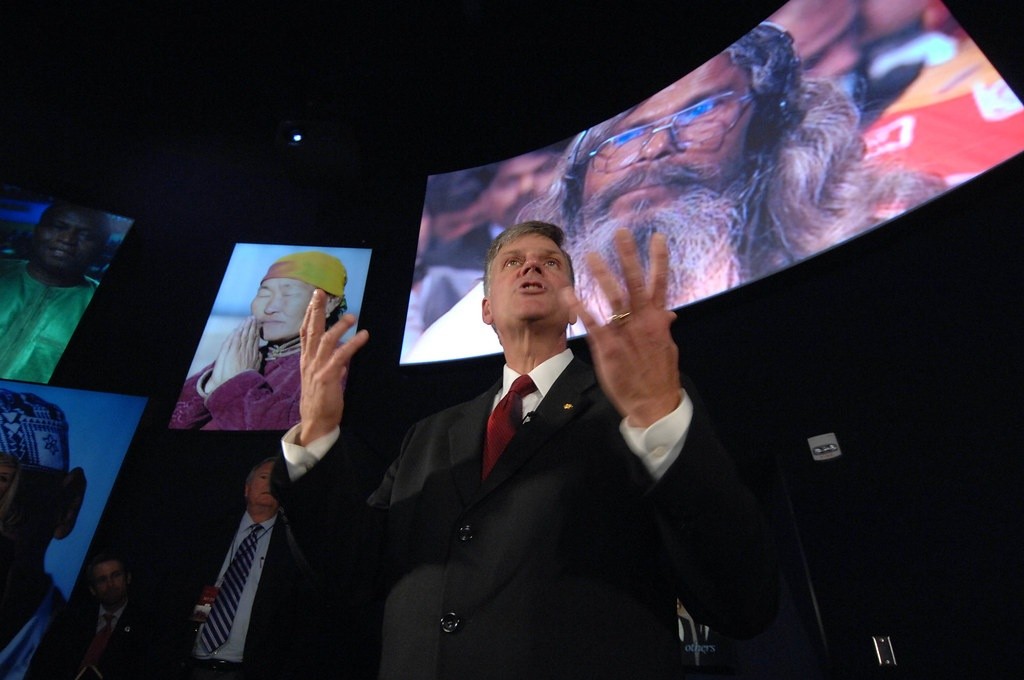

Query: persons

[0,393,86,680]
[0,205,112,385]
[169,252,351,430]
[21,551,161,680]
[268,223,731,680]
[171,456,332,680]
[400,19,947,367]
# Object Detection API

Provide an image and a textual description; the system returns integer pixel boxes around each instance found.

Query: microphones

[528,411,535,418]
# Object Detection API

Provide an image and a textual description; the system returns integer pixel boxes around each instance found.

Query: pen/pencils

[259,557,264,569]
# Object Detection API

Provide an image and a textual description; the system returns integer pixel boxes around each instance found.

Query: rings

[612,311,631,321]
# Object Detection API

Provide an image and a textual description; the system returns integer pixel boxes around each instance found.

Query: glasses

[578,88,753,173]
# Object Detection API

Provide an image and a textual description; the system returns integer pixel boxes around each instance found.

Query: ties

[481,375,539,484]
[193,523,264,655]
[79,611,116,674]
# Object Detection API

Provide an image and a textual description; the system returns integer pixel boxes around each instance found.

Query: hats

[0,389,70,473]
